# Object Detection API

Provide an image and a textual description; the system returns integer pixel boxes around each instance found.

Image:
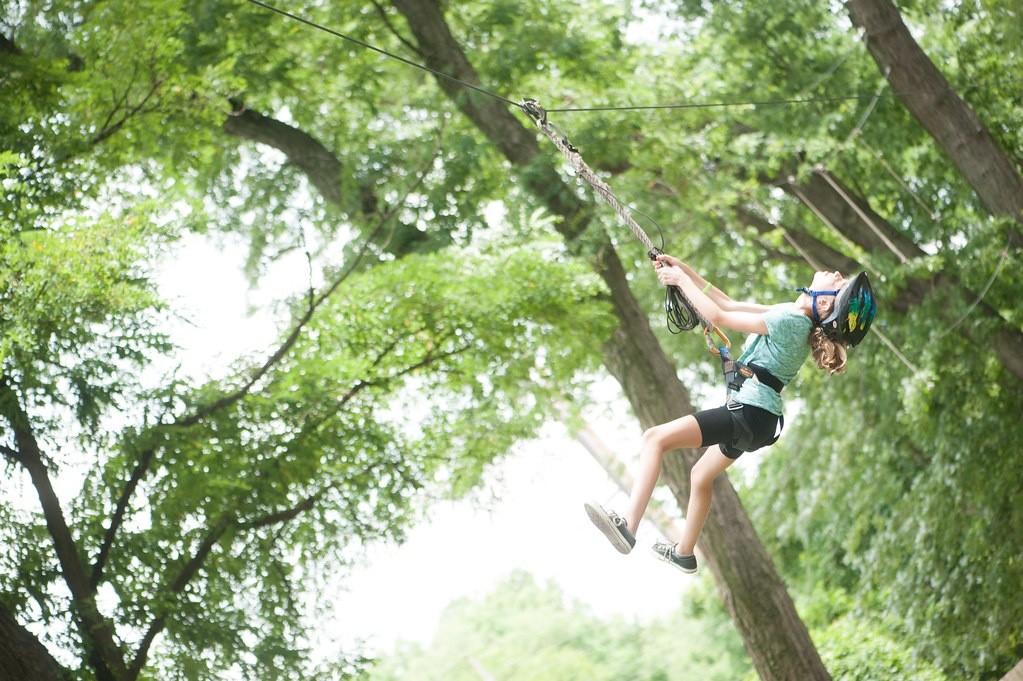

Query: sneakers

[650,538,697,574]
[584,501,637,554]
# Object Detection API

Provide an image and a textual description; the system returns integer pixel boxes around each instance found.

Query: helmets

[822,271,876,350]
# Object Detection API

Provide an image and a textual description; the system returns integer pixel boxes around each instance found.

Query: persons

[583,254,875,573]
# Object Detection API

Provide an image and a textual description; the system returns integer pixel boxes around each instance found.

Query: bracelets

[703,281,712,294]
